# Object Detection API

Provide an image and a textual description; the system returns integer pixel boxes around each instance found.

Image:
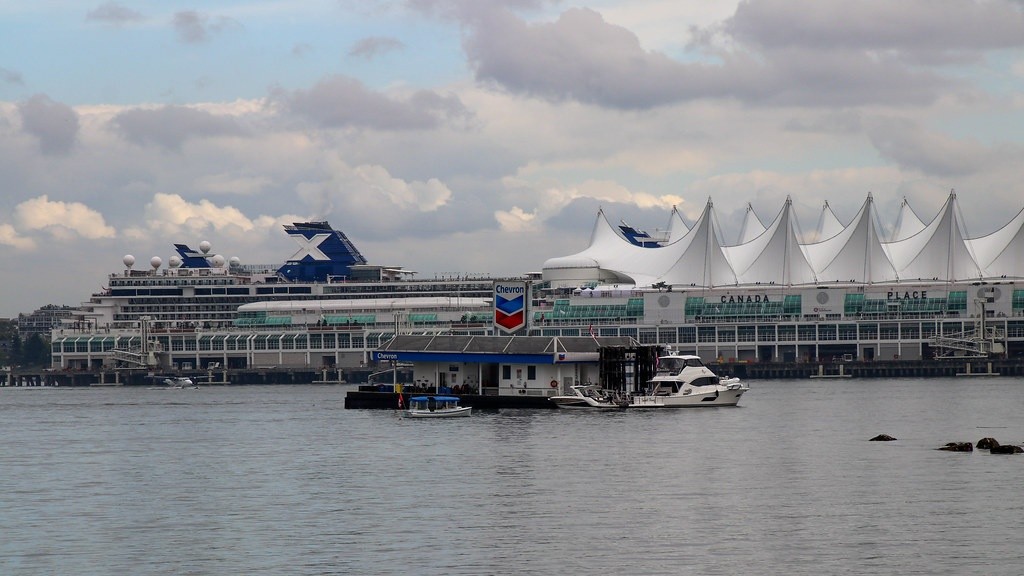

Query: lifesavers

[894,354,898,359]
[550,380,558,388]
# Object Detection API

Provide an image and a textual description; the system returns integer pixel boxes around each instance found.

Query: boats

[402,395,473,418]
[632,355,748,407]
[548,381,616,410]
[344,333,645,401]
